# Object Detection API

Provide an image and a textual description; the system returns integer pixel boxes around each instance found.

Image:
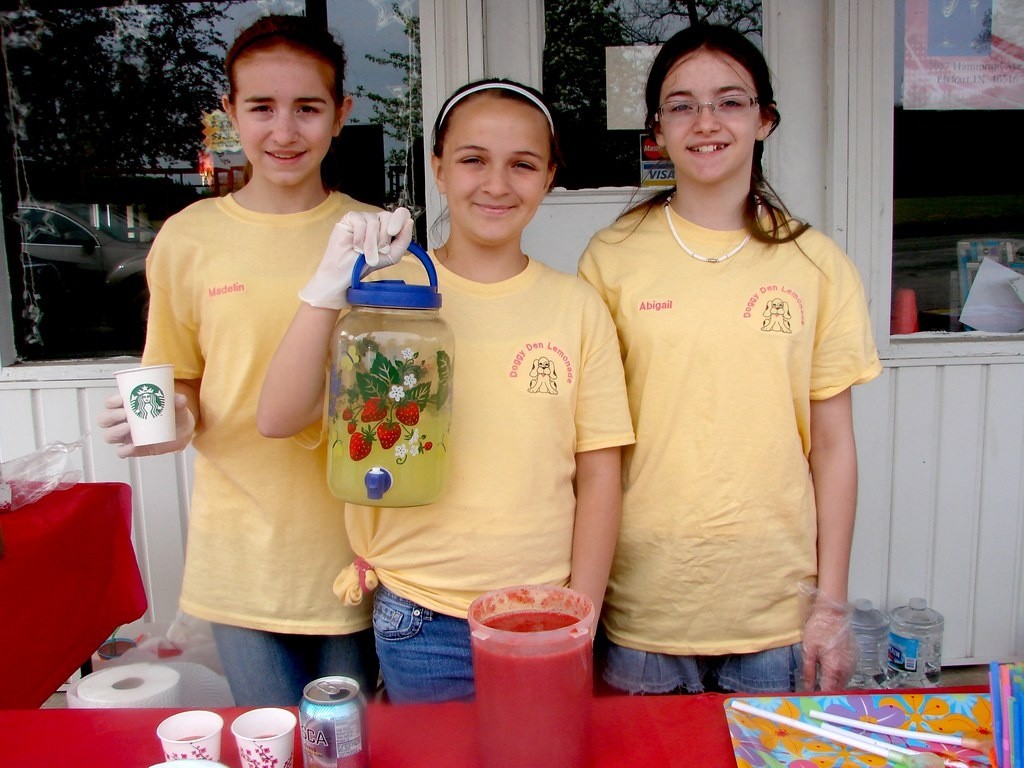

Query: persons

[577,23,882,693]
[100,13,409,707]
[256,79,635,703]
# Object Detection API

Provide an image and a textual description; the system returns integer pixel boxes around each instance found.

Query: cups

[467,583,595,768]
[230,707,297,768]
[157,710,224,760]
[114,365,177,446]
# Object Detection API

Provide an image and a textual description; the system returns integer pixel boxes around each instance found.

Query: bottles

[328,239,455,508]
[845,599,889,690]
[888,597,944,686]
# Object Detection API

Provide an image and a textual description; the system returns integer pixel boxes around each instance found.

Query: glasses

[652,95,762,122]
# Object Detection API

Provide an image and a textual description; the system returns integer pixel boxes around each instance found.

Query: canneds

[297,675,372,768]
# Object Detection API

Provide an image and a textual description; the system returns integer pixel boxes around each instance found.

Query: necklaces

[665,191,761,262]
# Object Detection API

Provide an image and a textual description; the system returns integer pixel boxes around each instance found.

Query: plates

[724,693,990,768]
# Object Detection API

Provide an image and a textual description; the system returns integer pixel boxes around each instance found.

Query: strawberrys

[342,398,433,460]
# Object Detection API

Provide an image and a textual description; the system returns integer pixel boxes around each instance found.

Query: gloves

[298,207,415,310]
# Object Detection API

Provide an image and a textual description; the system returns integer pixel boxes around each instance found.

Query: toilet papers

[65,660,235,709]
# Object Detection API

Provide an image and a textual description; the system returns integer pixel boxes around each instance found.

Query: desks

[0,480,993,768]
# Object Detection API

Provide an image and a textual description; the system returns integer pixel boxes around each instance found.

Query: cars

[15,201,173,354]
